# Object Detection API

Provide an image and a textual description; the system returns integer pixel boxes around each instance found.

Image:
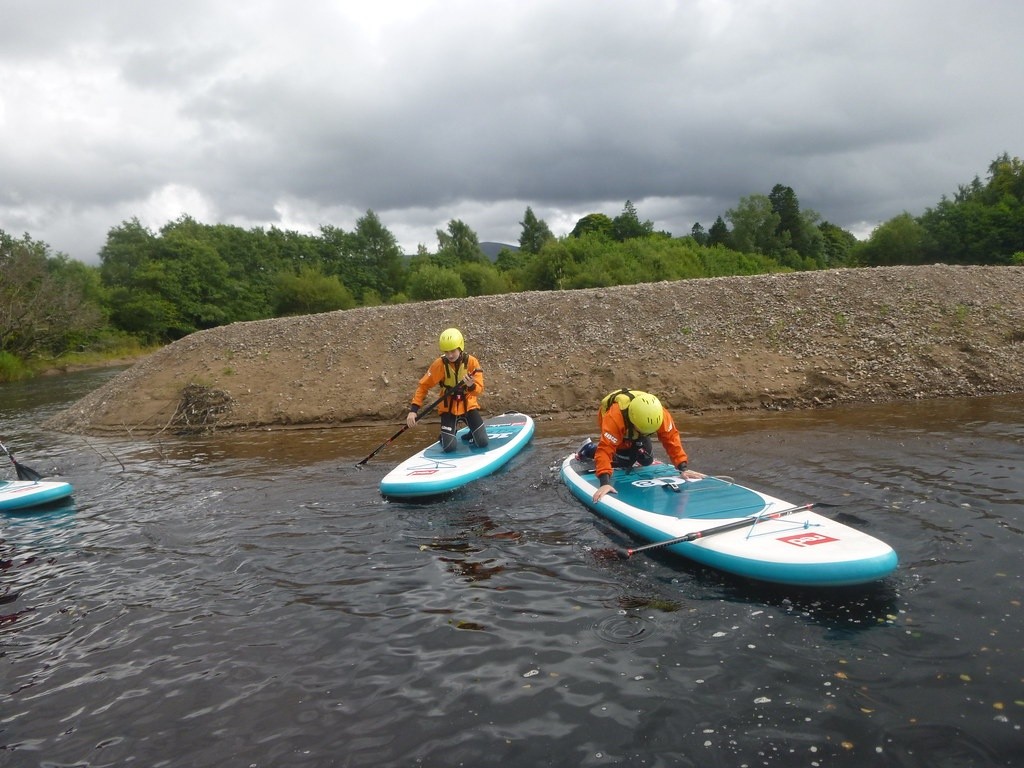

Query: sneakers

[575,437,594,461]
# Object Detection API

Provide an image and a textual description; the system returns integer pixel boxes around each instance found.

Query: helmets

[628,394,663,437]
[438,328,465,352]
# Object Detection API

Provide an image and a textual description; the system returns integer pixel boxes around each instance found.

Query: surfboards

[554,446,899,589]
[377,411,535,497]
[0,482,73,513]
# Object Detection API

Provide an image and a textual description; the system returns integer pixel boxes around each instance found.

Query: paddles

[351,369,488,469]
[0,439,39,477]
[610,497,855,563]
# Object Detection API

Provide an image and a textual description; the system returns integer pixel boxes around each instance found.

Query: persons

[575,389,707,503]
[406,329,489,452]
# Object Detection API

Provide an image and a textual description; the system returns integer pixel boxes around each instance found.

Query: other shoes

[461,430,474,443]
